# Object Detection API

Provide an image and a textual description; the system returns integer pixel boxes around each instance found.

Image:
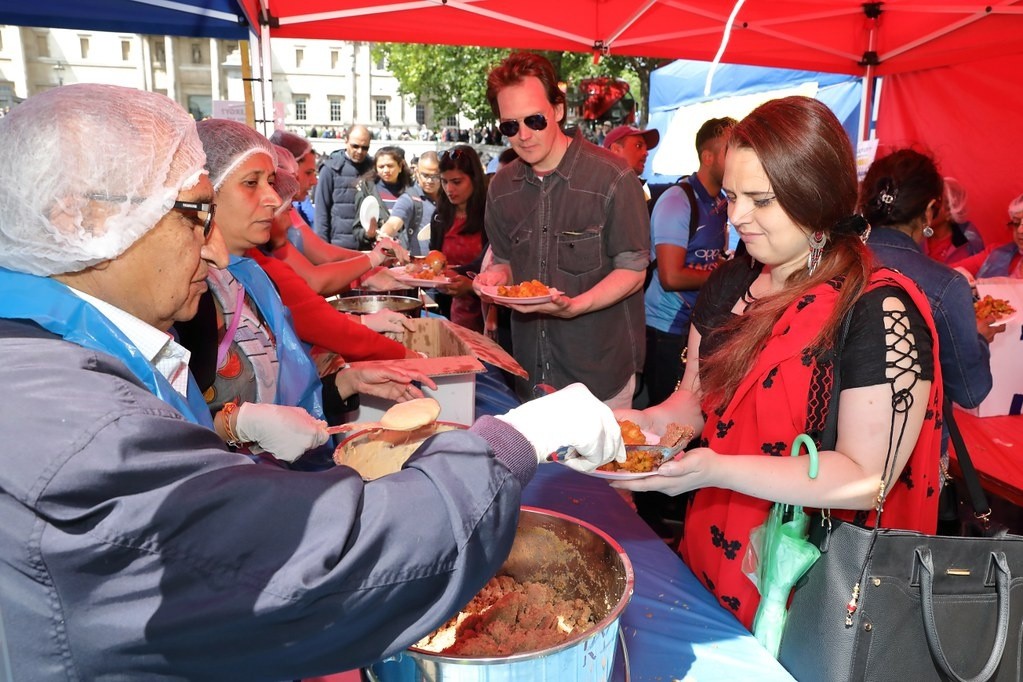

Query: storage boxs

[336,318,531,440]
[952,276,1023,417]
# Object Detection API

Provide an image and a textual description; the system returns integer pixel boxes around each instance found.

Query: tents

[0,0,1023,255]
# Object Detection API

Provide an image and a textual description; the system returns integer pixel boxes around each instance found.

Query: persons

[0,53,1023,682]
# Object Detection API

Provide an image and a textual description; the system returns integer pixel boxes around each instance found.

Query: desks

[944,404,1023,506]
[364,311,798,682]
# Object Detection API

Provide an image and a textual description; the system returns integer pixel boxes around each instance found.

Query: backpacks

[399,187,423,250]
[642,182,699,295]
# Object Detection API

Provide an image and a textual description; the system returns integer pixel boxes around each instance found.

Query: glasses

[350,144,369,150]
[80,193,218,240]
[499,112,547,137]
[419,173,440,179]
[1007,221,1023,231]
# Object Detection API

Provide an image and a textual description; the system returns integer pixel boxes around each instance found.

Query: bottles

[988,304,1017,326]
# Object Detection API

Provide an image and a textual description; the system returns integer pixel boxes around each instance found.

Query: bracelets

[220,399,240,448]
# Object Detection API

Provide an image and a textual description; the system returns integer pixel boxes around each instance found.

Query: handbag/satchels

[778,267,1023,682]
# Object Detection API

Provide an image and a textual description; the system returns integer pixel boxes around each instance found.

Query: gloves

[494,382,626,474]
[361,307,416,343]
[367,238,411,269]
[236,401,329,465]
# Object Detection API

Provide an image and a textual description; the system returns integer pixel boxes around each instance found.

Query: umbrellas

[750,433,822,662]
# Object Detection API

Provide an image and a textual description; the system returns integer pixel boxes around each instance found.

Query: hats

[603,126,659,150]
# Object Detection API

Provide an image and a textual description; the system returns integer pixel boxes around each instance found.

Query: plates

[565,445,673,480]
[359,195,380,230]
[389,267,450,286]
[481,286,565,304]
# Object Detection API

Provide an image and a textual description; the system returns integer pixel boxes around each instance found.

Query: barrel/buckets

[327,295,424,335]
[333,421,472,482]
[403,505,635,682]
